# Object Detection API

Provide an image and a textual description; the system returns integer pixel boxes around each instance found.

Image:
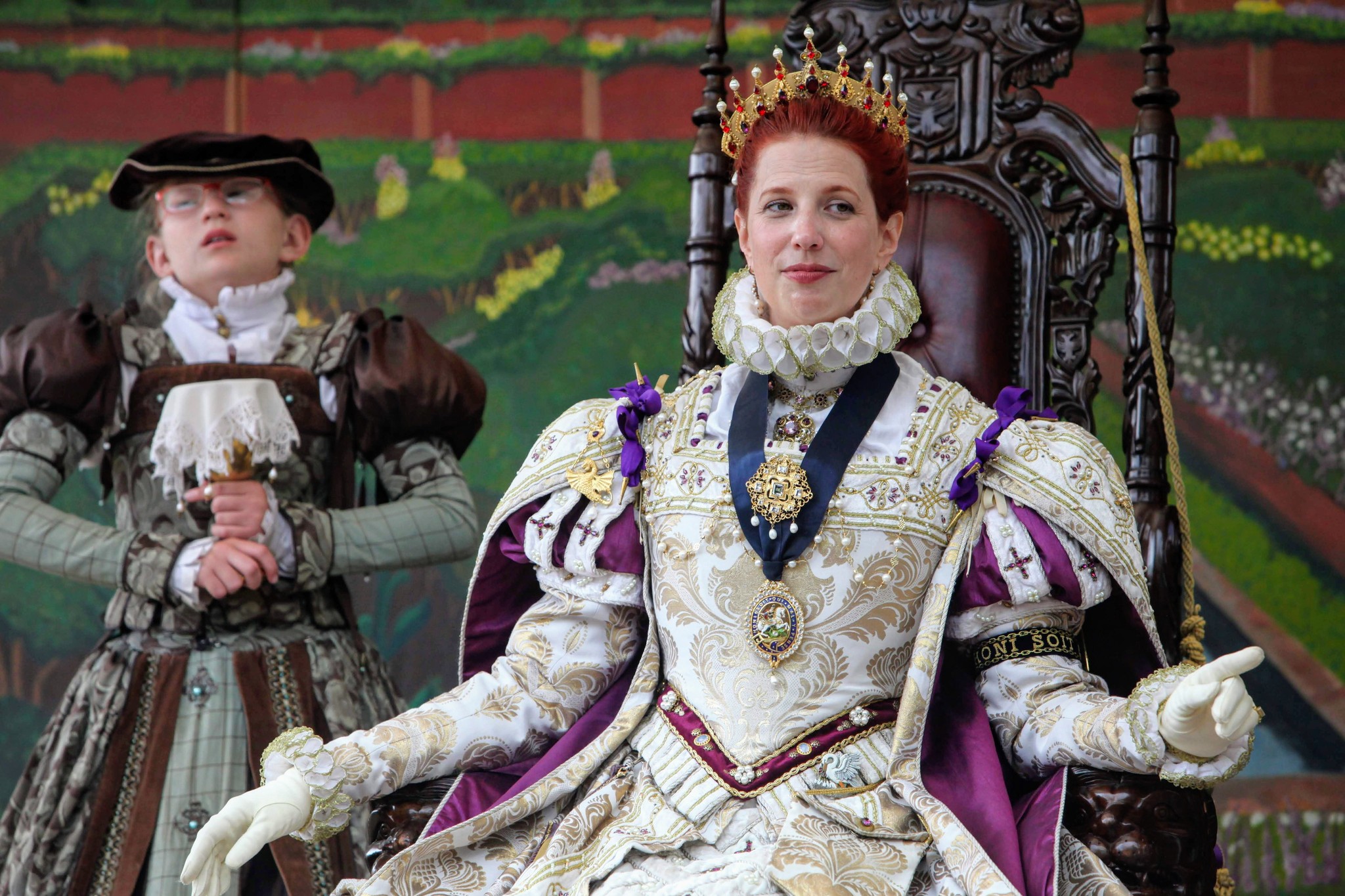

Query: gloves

[180,763,316,896]
[1157,646,1266,765]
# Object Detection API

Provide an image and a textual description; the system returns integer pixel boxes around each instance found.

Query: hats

[108,130,337,234]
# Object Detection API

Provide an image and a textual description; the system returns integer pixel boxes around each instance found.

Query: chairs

[366,1,1226,894]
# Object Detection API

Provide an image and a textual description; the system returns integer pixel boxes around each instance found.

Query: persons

[180,101,1259,896]
[0,131,483,896]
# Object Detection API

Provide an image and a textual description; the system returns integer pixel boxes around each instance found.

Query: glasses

[154,177,282,216]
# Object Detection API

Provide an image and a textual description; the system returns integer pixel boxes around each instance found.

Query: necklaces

[744,375,850,686]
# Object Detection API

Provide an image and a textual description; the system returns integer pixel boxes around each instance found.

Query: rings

[1251,704,1265,721]
